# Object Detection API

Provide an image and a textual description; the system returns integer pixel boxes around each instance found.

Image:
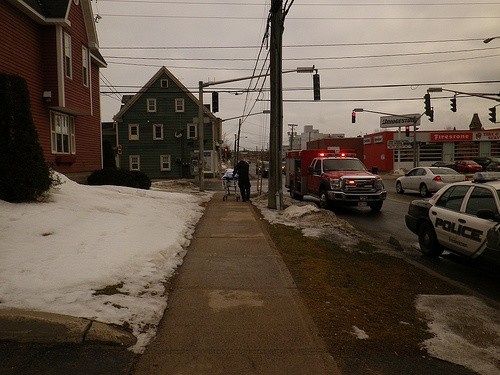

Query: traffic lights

[313,74,321,102]
[428,110,433,122]
[488,107,496,124]
[450,99,457,112]
[405,126,410,136]
[351,112,356,123]
[423,94,430,116]
[212,92,219,112]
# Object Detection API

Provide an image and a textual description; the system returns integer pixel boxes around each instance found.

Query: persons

[232,155,251,202]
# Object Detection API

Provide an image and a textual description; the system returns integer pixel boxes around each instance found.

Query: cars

[256,161,286,178]
[474,157,500,171]
[432,161,457,170]
[405,171,500,264]
[395,167,469,197]
[455,161,484,173]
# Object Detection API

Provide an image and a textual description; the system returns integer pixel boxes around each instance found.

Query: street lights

[288,124,298,150]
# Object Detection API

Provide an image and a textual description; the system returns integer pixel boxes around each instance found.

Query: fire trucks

[285,149,387,212]
